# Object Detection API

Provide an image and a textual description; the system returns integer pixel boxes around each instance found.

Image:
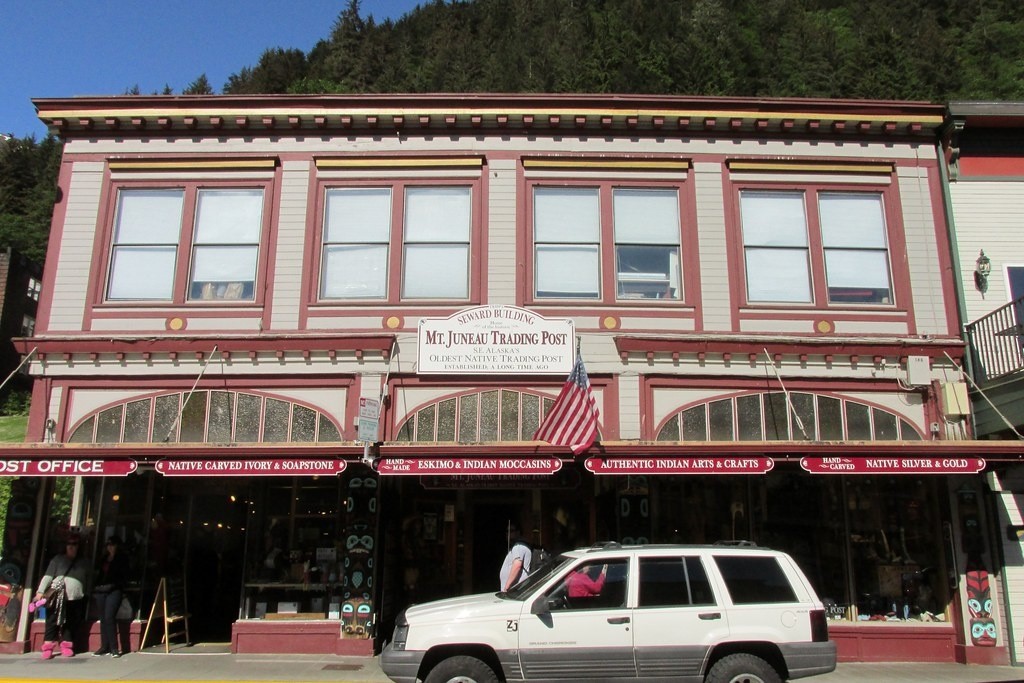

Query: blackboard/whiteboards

[149,577,187,620]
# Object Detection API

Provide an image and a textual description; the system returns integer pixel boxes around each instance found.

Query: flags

[533,357,600,455]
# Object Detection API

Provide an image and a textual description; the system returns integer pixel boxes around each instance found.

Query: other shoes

[111,648,120,658]
[40,643,54,659]
[93,646,110,656]
[60,642,73,657]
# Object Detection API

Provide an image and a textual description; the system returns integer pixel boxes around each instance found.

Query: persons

[34,535,92,659]
[499,522,532,591]
[96,535,133,658]
[565,563,608,597]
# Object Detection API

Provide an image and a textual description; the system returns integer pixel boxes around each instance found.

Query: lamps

[975,247,992,285]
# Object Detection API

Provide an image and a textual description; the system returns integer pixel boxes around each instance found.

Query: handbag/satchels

[41,587,57,609]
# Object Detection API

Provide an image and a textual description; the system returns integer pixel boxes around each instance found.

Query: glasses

[505,528,518,534]
[67,541,80,546]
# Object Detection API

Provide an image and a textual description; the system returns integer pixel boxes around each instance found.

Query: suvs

[376,541,837,683]
